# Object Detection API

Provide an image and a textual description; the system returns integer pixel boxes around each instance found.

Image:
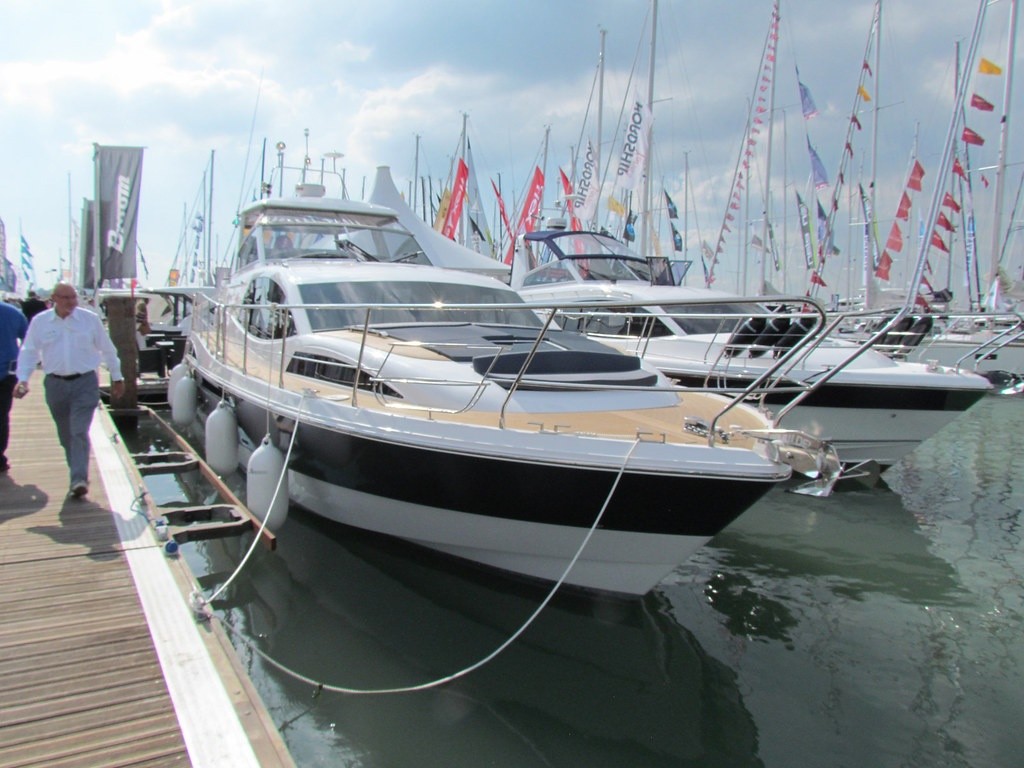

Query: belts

[49,373,95,380]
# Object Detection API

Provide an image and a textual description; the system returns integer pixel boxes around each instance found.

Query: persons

[12,280,126,499]
[0,302,29,472]
[7,291,54,365]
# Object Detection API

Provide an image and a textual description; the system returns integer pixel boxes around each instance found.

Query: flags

[93,144,145,280]
[79,197,94,290]
[429,2,1003,310]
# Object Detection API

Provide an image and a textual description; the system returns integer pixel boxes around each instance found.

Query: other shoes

[69,482,88,499]
[0,455,9,471]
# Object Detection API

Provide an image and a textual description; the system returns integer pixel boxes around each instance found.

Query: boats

[179,166,843,606]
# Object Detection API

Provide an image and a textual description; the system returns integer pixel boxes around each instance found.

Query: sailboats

[111,0,1024,484]
